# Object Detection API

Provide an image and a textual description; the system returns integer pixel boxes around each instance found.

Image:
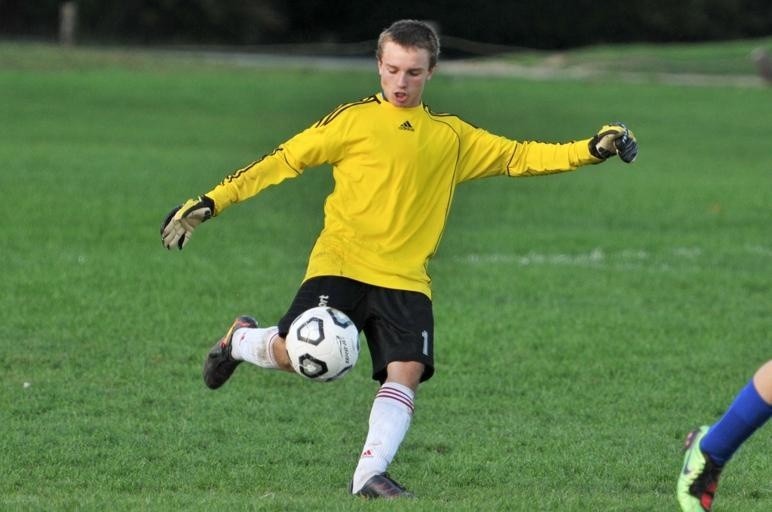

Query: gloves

[160,195,214,251]
[588,122,639,164]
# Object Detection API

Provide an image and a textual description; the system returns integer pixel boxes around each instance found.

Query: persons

[675,358,772,512]
[159,18,637,499]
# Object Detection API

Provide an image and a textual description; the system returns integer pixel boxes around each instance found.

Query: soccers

[286,307,360,383]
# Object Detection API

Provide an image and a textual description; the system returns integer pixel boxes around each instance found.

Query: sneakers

[678,425,722,512]
[348,472,413,499]
[203,315,258,390]
